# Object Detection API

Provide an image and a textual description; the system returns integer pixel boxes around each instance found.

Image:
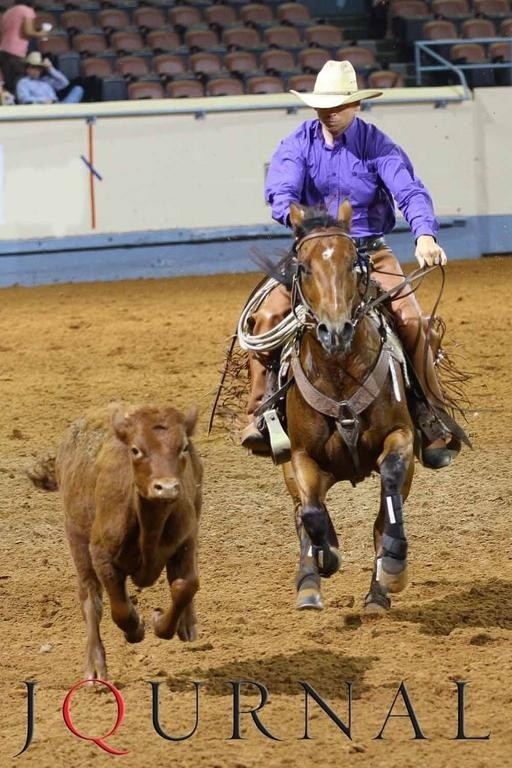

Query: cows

[23,400,204,688]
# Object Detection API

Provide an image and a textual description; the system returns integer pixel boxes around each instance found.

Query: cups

[40,23,52,41]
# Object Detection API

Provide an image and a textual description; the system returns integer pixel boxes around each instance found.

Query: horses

[248,197,417,613]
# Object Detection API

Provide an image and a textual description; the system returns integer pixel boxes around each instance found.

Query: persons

[240,62,452,469]
[0,1,51,90]
[17,52,84,105]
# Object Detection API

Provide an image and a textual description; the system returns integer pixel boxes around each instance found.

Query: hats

[289,58,383,109]
[19,50,48,68]
